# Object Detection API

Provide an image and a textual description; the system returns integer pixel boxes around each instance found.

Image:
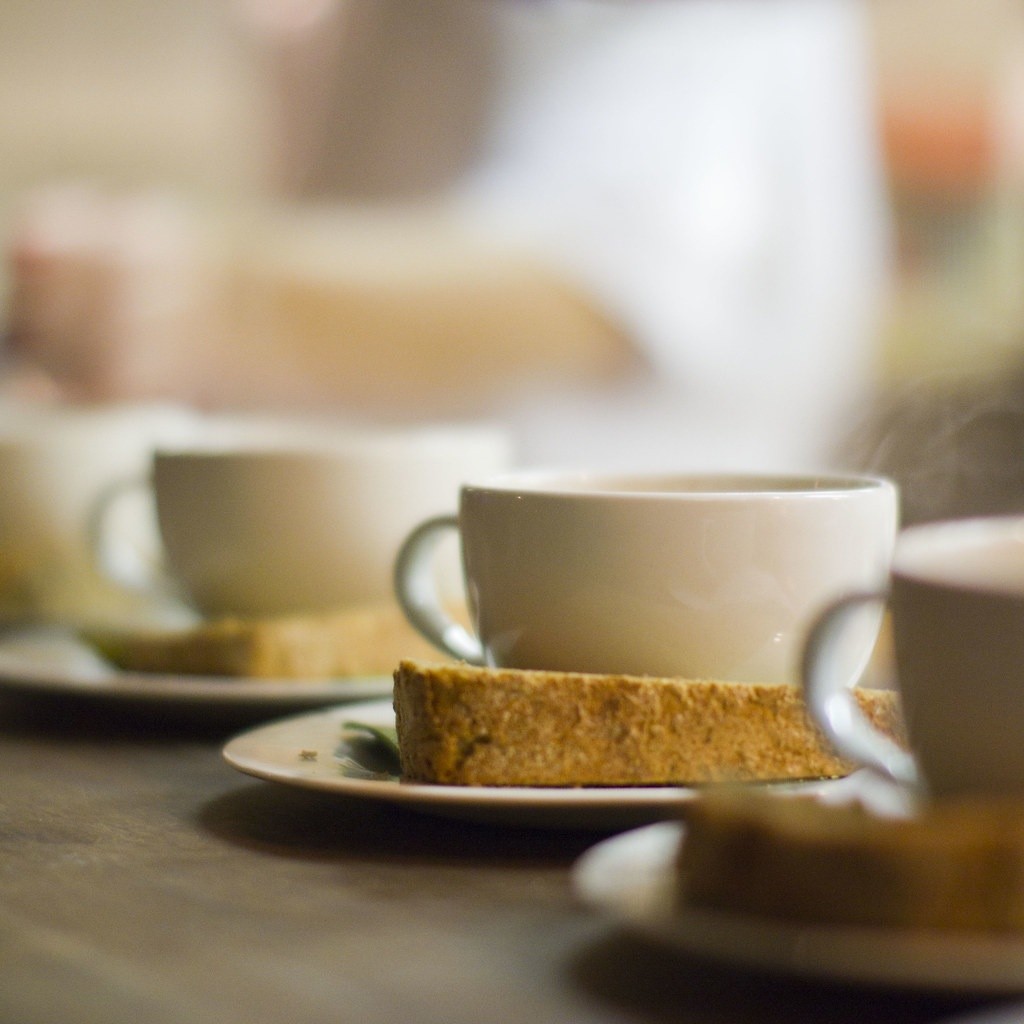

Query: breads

[392,662,908,789]
[84,597,469,678]
[675,789,1024,933]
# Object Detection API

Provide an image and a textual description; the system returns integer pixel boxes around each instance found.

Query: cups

[73,438,450,631]
[800,514,1024,805]
[391,466,902,692]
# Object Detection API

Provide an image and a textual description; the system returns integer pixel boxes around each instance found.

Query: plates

[566,816,1024,990]
[0,632,392,707]
[220,695,700,805]
